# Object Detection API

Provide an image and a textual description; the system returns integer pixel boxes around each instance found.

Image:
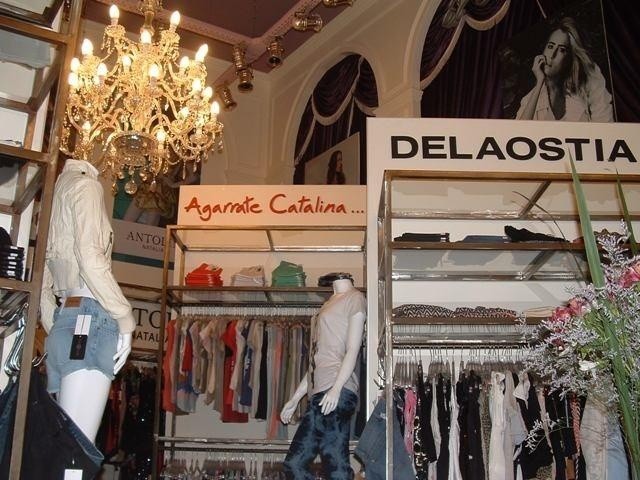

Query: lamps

[216,0,354,112]
[58,1,225,197]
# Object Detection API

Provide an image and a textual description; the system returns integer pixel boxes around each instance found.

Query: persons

[326,149,346,185]
[277,276,368,480]
[513,17,616,124]
[39,157,136,447]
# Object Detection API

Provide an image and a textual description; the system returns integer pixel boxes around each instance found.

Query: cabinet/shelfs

[0,0,82,480]
